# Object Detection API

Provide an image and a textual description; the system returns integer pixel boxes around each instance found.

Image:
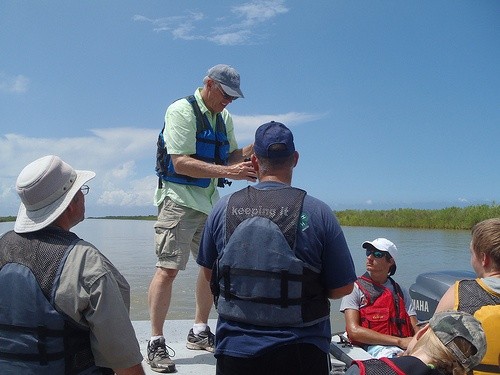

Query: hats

[361,238,399,264]
[207,64,244,98]
[14,155,96,233]
[429,310,487,371]
[254,120,296,159]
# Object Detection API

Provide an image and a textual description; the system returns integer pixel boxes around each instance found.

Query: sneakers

[147,336,175,373]
[186,326,216,351]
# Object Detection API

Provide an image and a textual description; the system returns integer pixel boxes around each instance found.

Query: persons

[0,155,146,375]
[196,121,357,375]
[346,311,487,375]
[398,218,500,375]
[340,238,420,358]
[146,64,257,373]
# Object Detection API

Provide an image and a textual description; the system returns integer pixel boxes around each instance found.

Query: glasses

[213,81,239,100]
[79,185,89,195]
[365,250,388,258]
